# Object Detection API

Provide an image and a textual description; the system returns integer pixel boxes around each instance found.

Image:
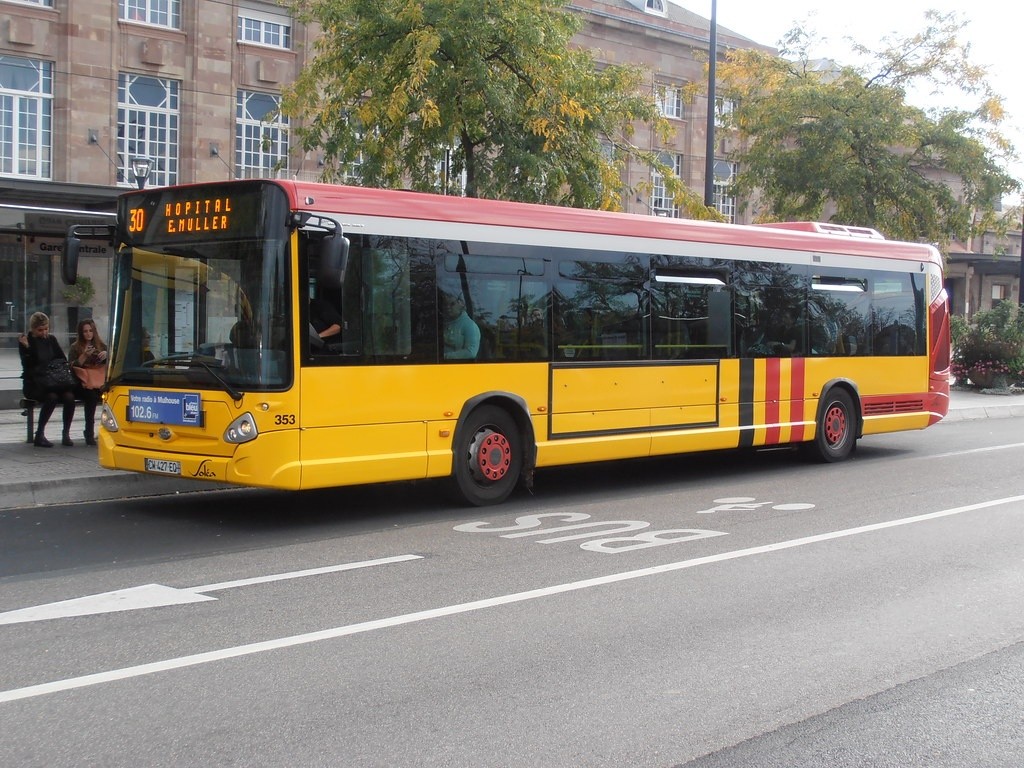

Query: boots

[33,430,54,446]
[62,430,74,446]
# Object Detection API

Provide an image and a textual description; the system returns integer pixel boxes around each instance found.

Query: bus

[61,178,951,508]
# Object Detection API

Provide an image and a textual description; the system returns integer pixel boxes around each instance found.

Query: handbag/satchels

[41,359,74,394]
[70,360,106,390]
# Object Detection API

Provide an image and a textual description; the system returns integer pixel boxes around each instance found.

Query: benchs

[19,398,102,444]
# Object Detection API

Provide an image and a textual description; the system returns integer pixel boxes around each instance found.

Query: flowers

[949,295,1023,384]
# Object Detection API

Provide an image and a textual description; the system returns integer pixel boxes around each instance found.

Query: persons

[18,311,76,448]
[444,291,481,360]
[142,327,161,368]
[68,318,108,446]
[308,296,342,355]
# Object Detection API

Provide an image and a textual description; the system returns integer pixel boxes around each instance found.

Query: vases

[968,368,1014,388]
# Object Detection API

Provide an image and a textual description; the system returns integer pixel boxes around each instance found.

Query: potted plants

[60,273,95,345]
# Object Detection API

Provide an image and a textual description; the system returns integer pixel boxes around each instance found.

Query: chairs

[324,297,365,354]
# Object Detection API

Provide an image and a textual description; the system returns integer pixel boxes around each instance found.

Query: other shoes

[84,430,96,445]
